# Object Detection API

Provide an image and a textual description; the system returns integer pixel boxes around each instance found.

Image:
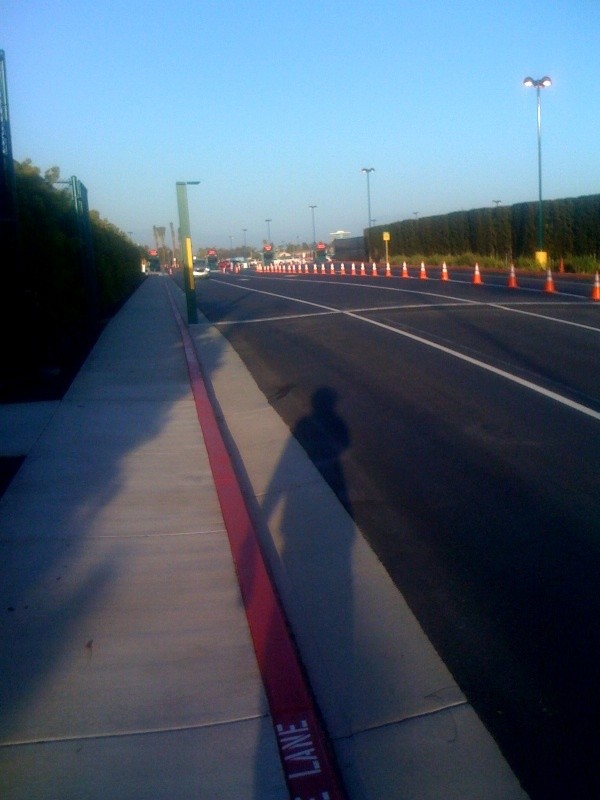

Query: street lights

[176,181,202,327]
[522,76,552,267]
[361,168,375,262]
[265,220,272,244]
[309,205,318,262]
[243,229,248,248]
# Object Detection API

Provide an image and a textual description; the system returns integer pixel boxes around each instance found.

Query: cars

[193,259,210,278]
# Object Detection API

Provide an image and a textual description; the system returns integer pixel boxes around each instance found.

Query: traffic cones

[350,261,357,276]
[590,270,599,303]
[417,261,428,280]
[544,267,559,294]
[385,261,392,277]
[360,262,367,276]
[340,262,346,276]
[401,261,410,279]
[472,262,485,286]
[304,264,311,275]
[440,261,450,281]
[256,262,297,274]
[297,263,303,274]
[506,263,520,290]
[330,263,336,275]
[321,263,326,275]
[313,264,318,275]
[371,262,378,277]
